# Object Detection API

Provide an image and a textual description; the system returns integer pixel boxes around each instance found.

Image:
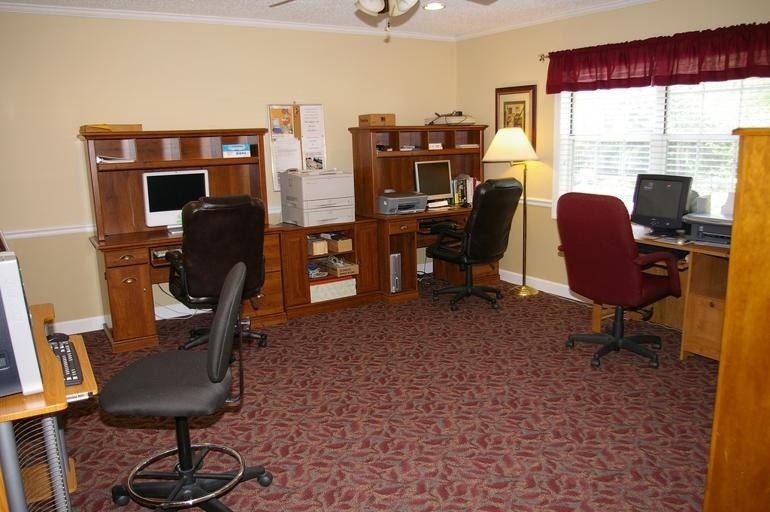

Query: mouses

[461,202,472,208]
[45,331,70,341]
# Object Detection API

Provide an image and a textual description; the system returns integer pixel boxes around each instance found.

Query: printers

[377,192,428,215]
[682,212,733,245]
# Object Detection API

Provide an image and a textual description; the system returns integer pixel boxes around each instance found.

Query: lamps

[353,0,422,44]
[481,127,541,296]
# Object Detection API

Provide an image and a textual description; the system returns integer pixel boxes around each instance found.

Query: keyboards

[46,340,83,386]
[419,220,458,227]
[153,248,182,258]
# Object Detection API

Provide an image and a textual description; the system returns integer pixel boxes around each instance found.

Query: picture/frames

[494,83,538,155]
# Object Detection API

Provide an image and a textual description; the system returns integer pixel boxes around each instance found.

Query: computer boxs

[389,251,403,293]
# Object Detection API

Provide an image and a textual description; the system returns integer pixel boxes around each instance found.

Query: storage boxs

[359,113,395,129]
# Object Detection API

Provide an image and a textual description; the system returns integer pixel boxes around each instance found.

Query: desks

[558,225,731,365]
[0,303,98,512]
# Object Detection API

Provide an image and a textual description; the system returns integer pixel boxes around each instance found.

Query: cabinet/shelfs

[348,122,488,304]
[279,216,382,318]
[702,126,770,511]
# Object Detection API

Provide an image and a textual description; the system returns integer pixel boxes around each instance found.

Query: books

[447,176,481,204]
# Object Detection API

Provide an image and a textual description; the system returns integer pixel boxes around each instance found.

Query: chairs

[428,176,524,312]
[165,195,268,352]
[555,191,683,370]
[96,260,274,512]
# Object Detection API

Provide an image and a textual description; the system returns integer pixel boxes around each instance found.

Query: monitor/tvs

[631,173,693,236]
[143,169,210,232]
[0,251,45,401]
[413,158,453,208]
